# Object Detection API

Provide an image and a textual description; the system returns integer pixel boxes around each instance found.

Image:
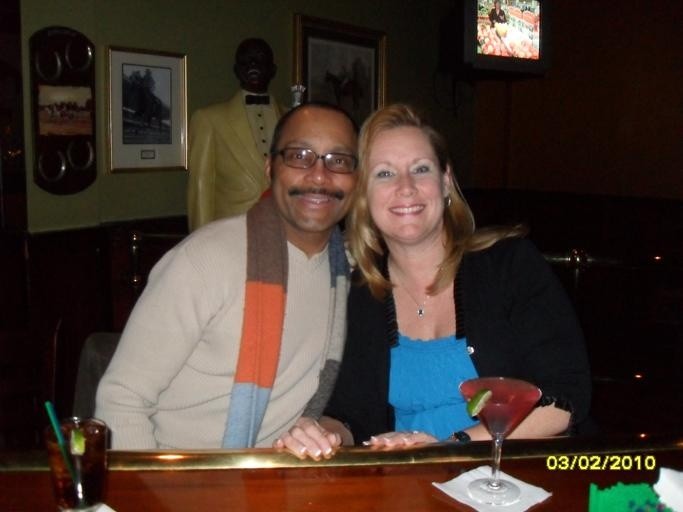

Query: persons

[184,36,292,236]
[488,0,507,29]
[267,103,596,464]
[90,101,361,453]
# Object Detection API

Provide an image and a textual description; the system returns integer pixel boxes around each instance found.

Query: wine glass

[459,375,544,506]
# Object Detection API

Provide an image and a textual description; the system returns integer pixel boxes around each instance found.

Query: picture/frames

[104,46,191,173]
[295,12,385,138]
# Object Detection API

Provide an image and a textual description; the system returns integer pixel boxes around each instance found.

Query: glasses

[267,146,360,174]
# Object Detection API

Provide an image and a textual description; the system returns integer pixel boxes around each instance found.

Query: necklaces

[389,258,430,320]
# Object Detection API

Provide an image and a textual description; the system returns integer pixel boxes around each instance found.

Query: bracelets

[452,428,470,443]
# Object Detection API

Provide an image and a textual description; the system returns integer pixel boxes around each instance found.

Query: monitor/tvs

[441,0,552,77]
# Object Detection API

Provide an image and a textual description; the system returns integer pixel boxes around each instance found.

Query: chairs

[130,228,189,310]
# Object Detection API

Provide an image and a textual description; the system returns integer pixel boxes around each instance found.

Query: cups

[42,416,108,512]
